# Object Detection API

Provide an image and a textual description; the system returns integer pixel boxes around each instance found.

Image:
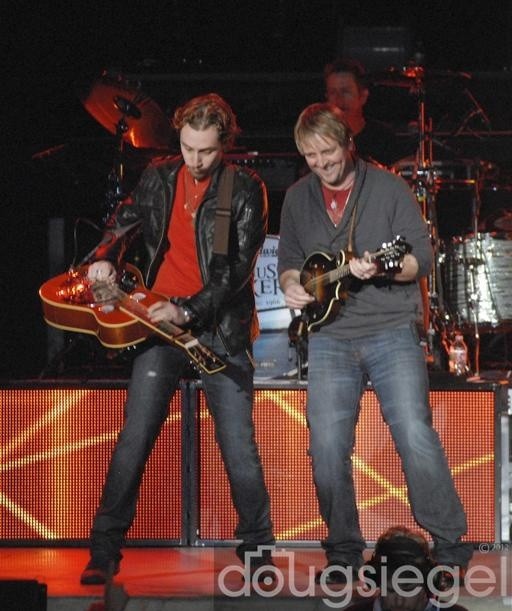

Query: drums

[441,230,512,333]
[431,177,512,235]
[396,162,491,177]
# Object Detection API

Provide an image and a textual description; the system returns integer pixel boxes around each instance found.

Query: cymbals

[83,81,168,149]
[377,65,472,91]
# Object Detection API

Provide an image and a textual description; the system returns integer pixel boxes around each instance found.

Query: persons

[75,93,278,590]
[280,101,475,586]
[337,527,471,611]
[295,54,400,183]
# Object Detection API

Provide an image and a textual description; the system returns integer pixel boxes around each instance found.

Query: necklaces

[330,177,355,212]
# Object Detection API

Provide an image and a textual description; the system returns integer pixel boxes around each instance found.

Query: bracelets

[180,305,193,322]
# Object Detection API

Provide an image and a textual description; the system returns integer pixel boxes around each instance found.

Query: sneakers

[432,562,466,587]
[80,557,119,584]
[241,560,274,581]
[314,561,356,583]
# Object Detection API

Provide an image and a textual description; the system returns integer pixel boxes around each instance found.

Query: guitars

[298,234,412,334]
[39,259,228,376]
